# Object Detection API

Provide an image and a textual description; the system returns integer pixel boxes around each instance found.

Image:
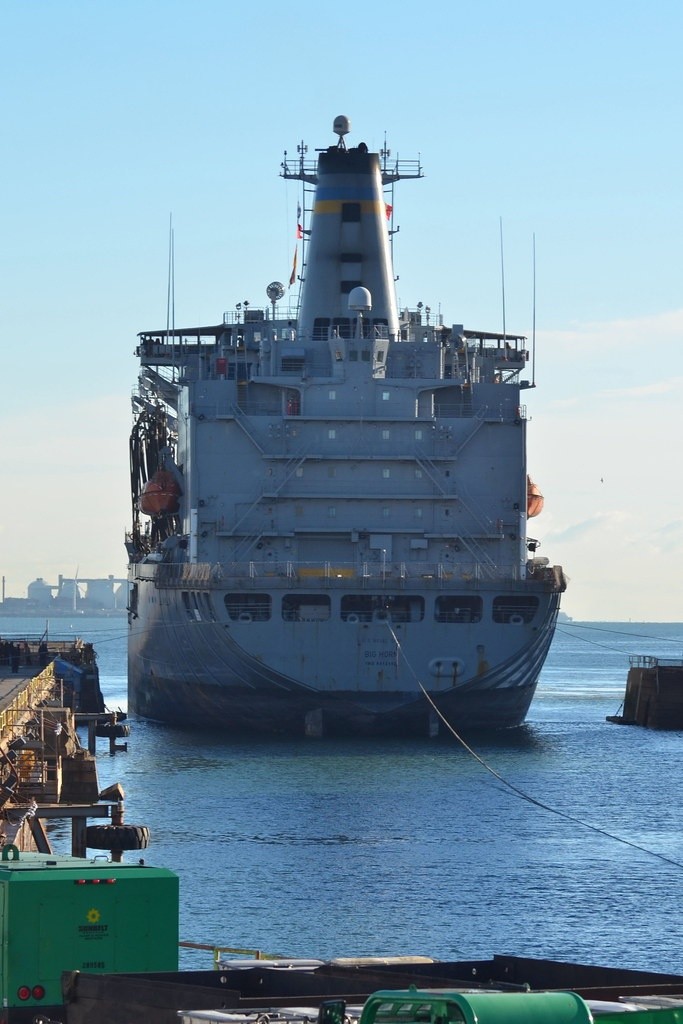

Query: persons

[0,641,49,674]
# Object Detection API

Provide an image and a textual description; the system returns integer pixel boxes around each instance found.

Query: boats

[123,105,566,738]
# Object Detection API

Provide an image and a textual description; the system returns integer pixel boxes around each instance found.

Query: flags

[385,203,393,222]
[289,247,298,285]
[296,224,303,239]
